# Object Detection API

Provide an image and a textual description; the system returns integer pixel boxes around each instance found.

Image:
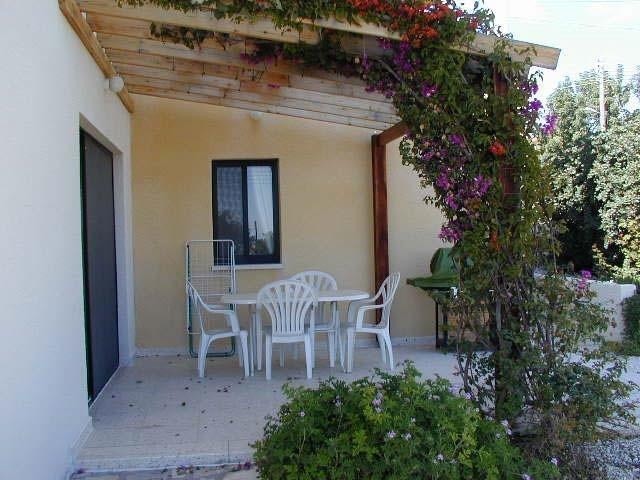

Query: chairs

[185,271,400,381]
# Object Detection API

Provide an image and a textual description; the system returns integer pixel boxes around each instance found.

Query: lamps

[250,111,263,121]
[102,77,124,95]
